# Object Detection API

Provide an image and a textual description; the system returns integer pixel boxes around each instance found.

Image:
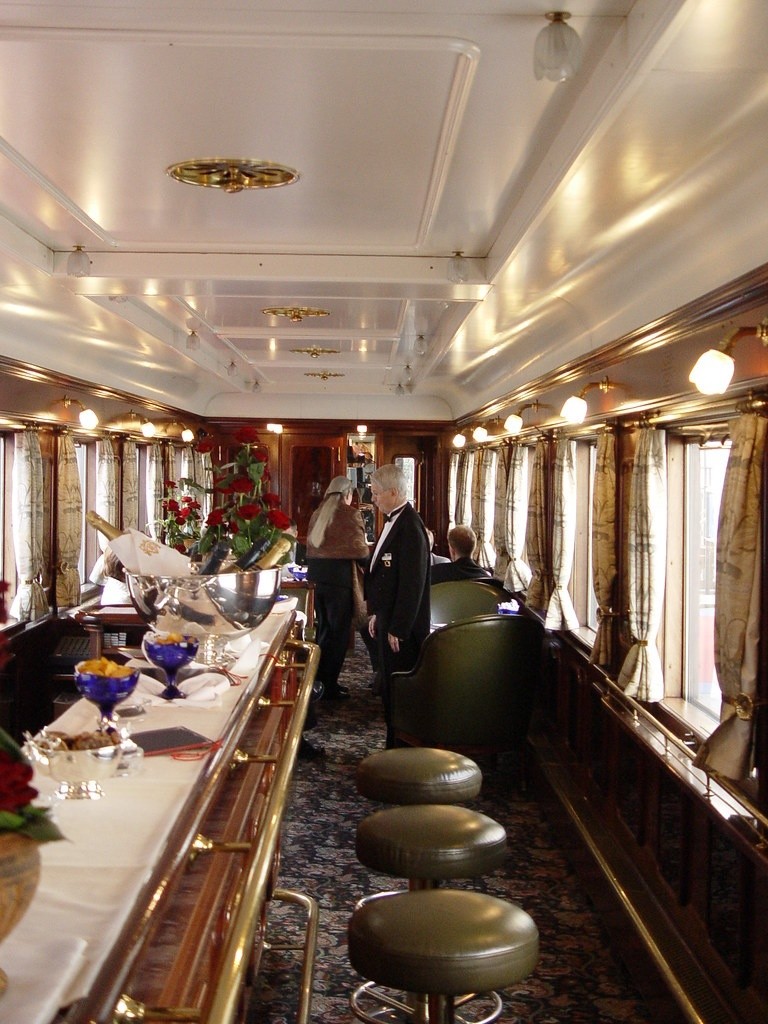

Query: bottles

[217,537,271,574]
[84,510,125,541]
[201,543,229,575]
[245,538,290,572]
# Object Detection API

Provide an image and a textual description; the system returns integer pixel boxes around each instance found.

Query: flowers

[159,426,298,566]
[0,725,72,843]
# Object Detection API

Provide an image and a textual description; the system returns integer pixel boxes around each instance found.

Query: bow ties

[382,503,406,523]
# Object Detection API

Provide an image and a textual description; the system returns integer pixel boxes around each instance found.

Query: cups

[193,632,229,665]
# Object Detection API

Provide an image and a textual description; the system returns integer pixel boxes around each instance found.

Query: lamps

[453,375,630,447]
[253,380,264,392]
[65,245,90,275]
[533,10,586,80]
[124,408,157,436]
[414,334,430,356]
[395,385,406,398]
[55,393,99,430]
[689,316,768,394]
[226,362,239,378]
[400,364,415,381]
[166,419,195,442]
[446,251,468,280]
[186,318,202,350]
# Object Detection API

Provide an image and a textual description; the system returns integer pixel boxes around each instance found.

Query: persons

[363,464,431,750]
[430,525,490,585]
[363,452,373,463]
[425,527,434,552]
[305,476,369,703]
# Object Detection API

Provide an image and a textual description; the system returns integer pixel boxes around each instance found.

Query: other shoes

[319,685,351,700]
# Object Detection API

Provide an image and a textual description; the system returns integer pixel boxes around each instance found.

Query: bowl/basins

[121,566,282,643]
[288,567,307,579]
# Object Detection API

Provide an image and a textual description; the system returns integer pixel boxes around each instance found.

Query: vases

[1,826,44,994]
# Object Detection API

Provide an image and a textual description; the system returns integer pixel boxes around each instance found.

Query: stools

[347,890,541,1023]
[353,748,483,919]
[350,806,506,1024]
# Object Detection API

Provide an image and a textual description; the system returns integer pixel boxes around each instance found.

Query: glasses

[371,487,397,496]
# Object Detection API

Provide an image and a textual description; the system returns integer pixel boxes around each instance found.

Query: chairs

[386,581,543,792]
